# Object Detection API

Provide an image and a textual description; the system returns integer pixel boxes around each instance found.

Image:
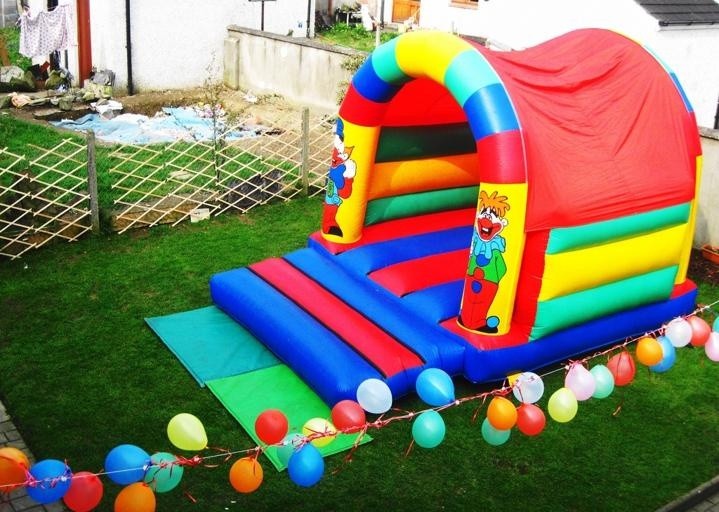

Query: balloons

[415,367,455,407]
[0,448,30,492]
[228,457,265,493]
[331,400,366,432]
[304,417,340,449]
[63,471,104,511]
[166,412,209,452]
[113,481,156,512]
[276,433,310,466]
[27,459,72,503]
[412,409,447,448]
[256,408,288,445]
[288,443,326,488]
[145,451,184,494]
[356,377,393,415]
[104,443,153,485]
[481,304,719,446]
